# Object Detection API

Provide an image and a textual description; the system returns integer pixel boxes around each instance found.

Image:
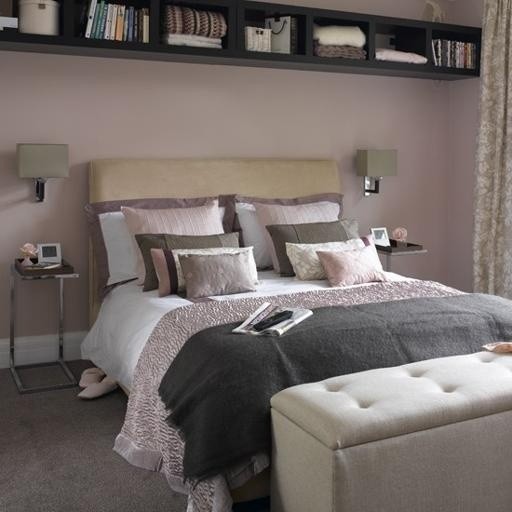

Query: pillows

[117,198,224,285]
[251,203,338,273]
[286,234,375,281]
[317,245,386,288]
[149,248,259,297]
[178,250,256,297]
[265,216,361,276]
[134,232,240,291]
[85,194,236,295]
[229,202,340,270]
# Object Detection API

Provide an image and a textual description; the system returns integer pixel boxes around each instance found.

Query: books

[432,39,477,69]
[85,0,148,43]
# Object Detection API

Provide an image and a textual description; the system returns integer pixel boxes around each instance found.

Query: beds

[84,157,512,511]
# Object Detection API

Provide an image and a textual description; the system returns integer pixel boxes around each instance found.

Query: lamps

[17,143,70,202]
[357,148,398,196]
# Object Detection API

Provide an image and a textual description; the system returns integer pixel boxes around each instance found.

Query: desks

[8,258,81,394]
[373,239,428,270]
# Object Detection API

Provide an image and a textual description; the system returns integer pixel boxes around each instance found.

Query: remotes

[253,309,294,331]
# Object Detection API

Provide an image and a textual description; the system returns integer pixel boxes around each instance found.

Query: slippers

[78,367,104,388]
[76,376,118,399]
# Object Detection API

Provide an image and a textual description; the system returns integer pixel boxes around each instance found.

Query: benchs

[270,349,512,511]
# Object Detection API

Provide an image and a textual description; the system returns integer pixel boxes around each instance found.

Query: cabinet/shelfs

[1,0,484,79]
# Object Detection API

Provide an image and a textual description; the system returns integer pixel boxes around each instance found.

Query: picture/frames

[37,243,62,263]
[370,227,391,246]
